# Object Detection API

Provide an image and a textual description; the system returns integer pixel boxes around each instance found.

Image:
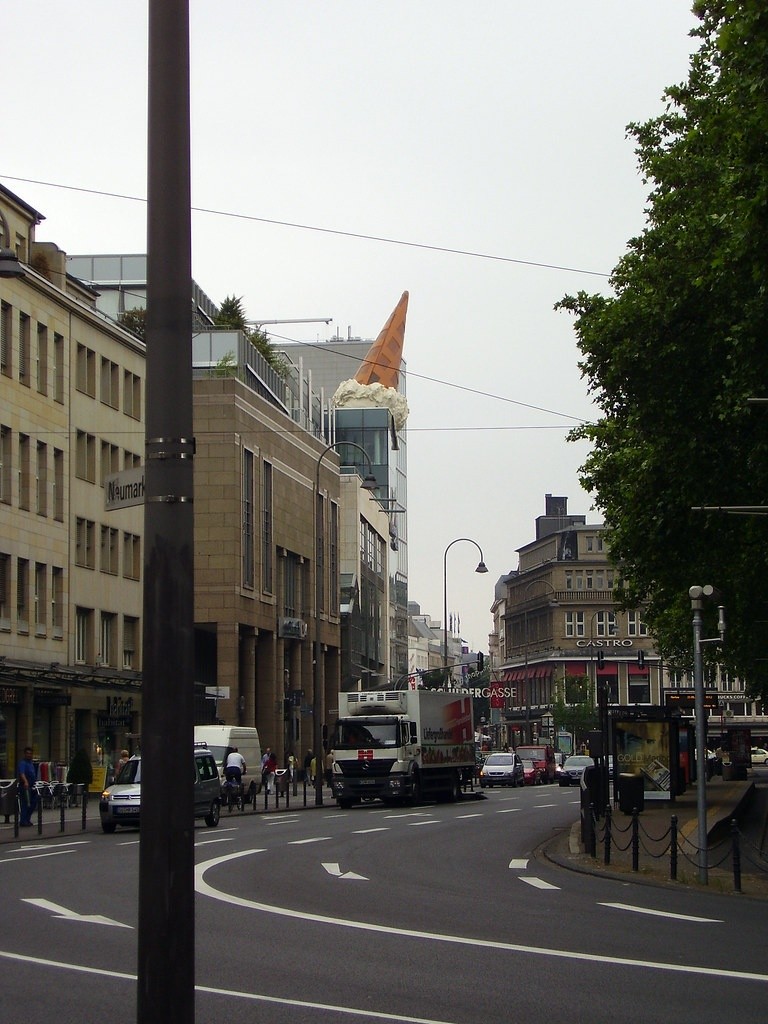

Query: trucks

[323,689,476,809]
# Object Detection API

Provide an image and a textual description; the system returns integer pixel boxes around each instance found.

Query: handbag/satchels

[262,765,270,775]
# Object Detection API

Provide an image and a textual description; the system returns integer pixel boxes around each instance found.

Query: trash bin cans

[275,769,289,791]
[0,779,19,824]
[722,761,732,780]
[618,773,645,815]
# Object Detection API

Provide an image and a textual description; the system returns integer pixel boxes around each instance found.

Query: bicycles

[226,774,243,811]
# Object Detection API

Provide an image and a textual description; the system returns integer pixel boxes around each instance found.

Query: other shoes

[20,820,34,827]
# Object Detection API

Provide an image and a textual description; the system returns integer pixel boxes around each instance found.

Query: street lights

[590,610,623,764]
[315,439,380,805]
[524,579,560,743]
[445,538,490,691]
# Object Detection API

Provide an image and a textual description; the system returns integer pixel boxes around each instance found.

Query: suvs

[100,741,223,833]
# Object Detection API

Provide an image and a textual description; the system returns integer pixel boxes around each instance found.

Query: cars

[559,755,595,786]
[694,748,719,762]
[599,754,613,780]
[479,753,525,787]
[520,759,542,786]
[751,748,768,765]
[475,757,486,776]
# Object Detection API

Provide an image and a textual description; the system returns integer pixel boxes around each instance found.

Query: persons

[18,747,38,826]
[716,744,723,776]
[116,750,130,776]
[225,747,246,801]
[503,743,509,752]
[482,742,488,751]
[580,741,586,755]
[261,748,334,795]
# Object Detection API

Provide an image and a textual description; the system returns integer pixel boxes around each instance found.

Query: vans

[194,724,267,802]
[554,752,566,781]
[516,746,556,784]
